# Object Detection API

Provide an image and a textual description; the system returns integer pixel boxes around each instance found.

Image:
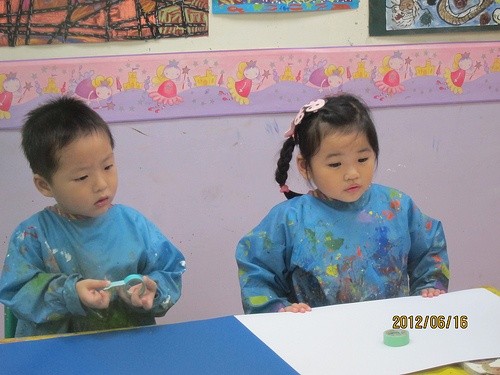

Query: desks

[0,285,499,374]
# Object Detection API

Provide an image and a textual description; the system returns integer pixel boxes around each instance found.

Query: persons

[236,91,449,317]
[1,95,188,340]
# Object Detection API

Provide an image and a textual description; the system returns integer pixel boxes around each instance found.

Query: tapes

[383,328,409,347]
[122,274,146,298]
